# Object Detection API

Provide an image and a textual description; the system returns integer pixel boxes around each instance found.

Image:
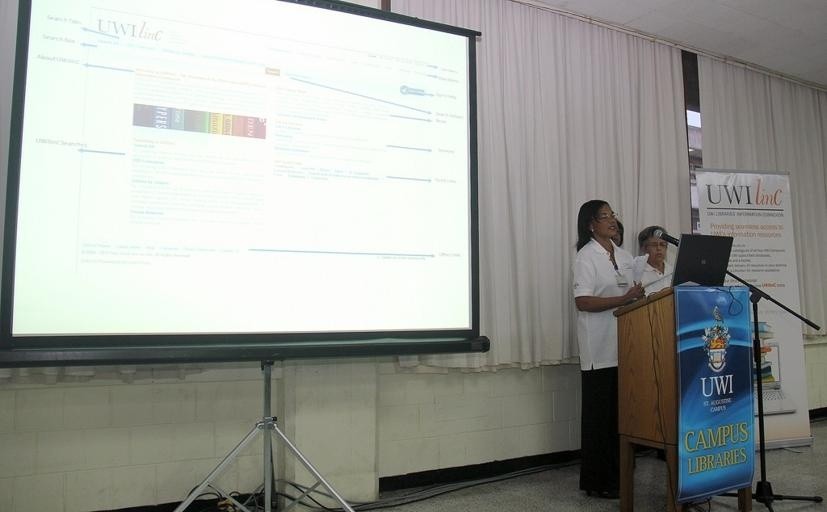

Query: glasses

[595,211,619,222]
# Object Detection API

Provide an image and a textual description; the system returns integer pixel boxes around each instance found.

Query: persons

[638,226,675,461]
[572,200,645,499]
[611,221,624,248]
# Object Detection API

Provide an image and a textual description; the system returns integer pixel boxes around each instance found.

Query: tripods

[718,270,822,512]
[171,361,354,512]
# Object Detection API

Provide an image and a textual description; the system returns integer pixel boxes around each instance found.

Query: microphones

[653,230,679,248]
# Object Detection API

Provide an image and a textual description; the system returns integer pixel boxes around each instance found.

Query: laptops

[671,234,734,290]
